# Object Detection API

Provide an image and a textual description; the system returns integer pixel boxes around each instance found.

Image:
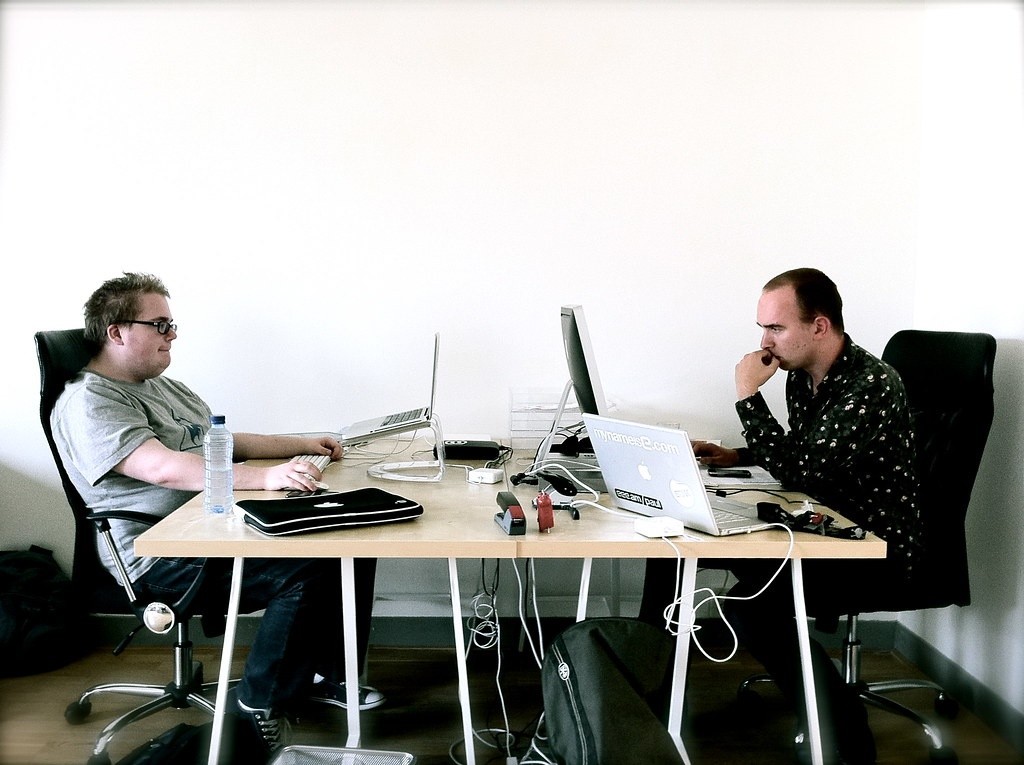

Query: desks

[503,448,887,765]
[134,444,515,765]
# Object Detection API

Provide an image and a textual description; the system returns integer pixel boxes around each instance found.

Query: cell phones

[708,468,752,478]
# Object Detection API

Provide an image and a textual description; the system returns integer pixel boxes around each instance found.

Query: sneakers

[311,675,387,712]
[225,686,293,765]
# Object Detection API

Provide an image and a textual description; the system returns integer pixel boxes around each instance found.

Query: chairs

[733,329,1001,765]
[36,327,254,764]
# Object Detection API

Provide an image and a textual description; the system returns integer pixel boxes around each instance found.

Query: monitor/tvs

[535,303,610,472]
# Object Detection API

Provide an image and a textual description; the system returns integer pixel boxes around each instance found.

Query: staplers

[494,492,526,535]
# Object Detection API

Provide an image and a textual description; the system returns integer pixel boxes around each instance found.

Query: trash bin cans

[271,744,419,765]
[0,548,70,677]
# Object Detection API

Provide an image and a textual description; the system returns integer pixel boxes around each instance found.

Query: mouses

[312,481,329,490]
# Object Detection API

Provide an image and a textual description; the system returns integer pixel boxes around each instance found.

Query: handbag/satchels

[0,544,103,680]
[542,618,695,765]
[116,722,205,765]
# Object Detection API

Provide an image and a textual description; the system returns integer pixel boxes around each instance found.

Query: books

[700,465,782,491]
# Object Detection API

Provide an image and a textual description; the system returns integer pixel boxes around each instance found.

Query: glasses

[104,319,178,335]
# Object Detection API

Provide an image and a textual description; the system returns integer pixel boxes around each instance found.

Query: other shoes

[824,677,877,765]
[691,689,793,751]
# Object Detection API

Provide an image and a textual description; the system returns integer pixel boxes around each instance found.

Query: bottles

[203,416,233,514]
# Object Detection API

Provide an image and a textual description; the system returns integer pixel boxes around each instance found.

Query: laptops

[582,413,778,536]
[342,331,440,440]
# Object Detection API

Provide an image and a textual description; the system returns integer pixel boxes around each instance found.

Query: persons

[687,267,925,765]
[50,273,384,765]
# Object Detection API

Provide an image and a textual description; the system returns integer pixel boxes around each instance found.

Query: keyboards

[290,454,331,473]
